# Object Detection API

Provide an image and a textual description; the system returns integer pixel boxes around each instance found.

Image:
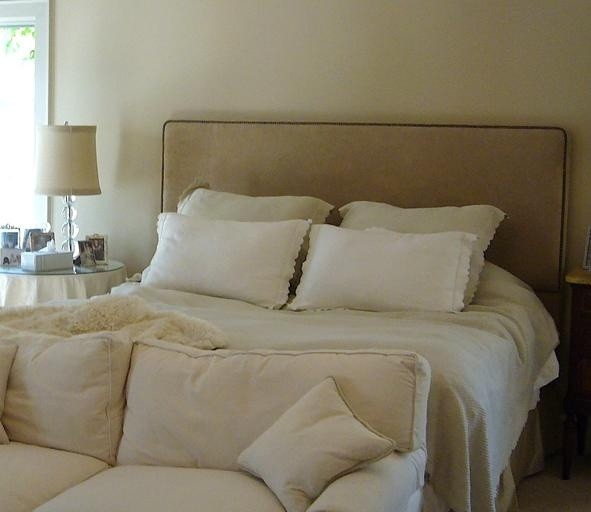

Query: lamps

[34,124,102,252]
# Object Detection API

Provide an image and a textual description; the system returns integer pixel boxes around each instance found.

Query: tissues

[21,239,74,273]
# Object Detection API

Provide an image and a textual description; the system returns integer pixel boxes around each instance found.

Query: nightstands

[562,267,591,482]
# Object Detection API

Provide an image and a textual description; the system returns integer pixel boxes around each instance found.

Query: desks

[0,256,128,304]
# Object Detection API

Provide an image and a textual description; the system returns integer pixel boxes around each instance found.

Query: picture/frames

[29,231,55,251]
[85,232,109,267]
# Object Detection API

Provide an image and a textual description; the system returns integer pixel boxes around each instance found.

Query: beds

[0,123,569,512]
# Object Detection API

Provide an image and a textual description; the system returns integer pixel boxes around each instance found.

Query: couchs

[0,326,434,512]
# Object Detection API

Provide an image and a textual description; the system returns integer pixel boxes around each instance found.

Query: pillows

[177,188,335,287]
[237,377,400,512]
[287,224,479,313]
[339,201,507,306]
[141,212,313,311]
